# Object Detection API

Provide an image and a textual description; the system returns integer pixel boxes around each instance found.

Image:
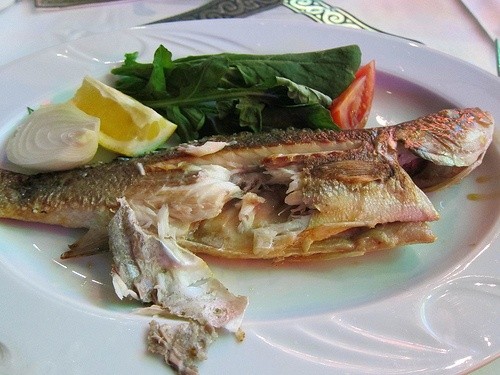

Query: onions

[4,102,100,170]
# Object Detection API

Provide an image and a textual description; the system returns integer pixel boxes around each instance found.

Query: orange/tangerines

[67,76,178,158]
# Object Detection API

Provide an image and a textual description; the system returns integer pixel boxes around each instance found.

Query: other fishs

[0,105,495,260]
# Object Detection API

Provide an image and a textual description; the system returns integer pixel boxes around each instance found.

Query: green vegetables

[109,44,361,144]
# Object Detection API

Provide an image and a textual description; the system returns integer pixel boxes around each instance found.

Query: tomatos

[328,60,377,131]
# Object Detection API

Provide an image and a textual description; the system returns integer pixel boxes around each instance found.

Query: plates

[1,18,500,374]
